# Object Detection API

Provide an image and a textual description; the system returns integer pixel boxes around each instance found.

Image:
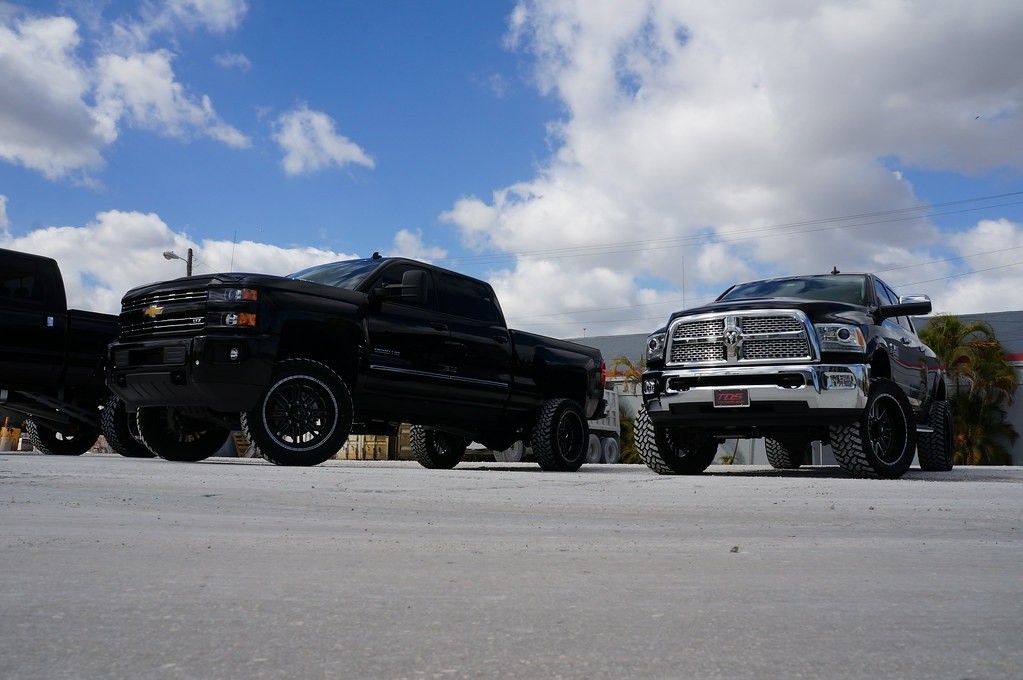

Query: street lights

[163,247,194,277]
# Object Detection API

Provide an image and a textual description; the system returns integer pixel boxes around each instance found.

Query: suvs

[633,264,957,482]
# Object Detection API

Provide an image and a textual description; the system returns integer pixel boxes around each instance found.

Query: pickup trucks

[0,246,158,458]
[103,248,607,473]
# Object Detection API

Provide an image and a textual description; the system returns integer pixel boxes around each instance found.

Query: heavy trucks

[465,385,620,462]
